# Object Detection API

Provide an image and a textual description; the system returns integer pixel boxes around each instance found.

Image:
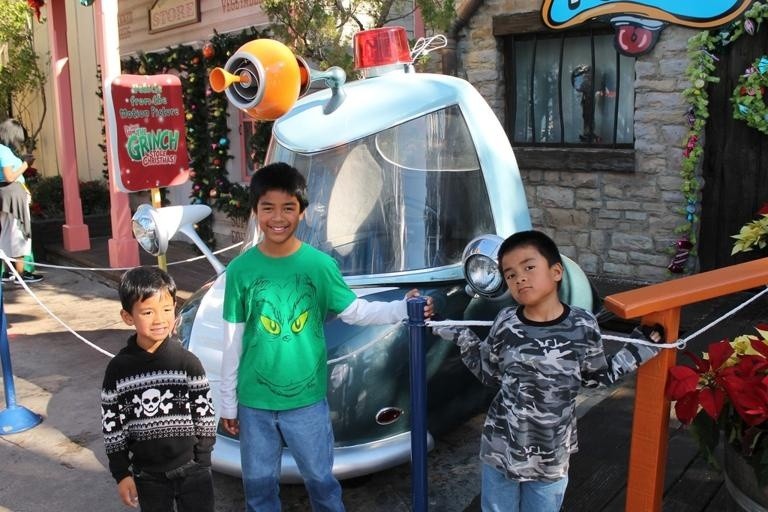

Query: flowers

[668,326,768,431]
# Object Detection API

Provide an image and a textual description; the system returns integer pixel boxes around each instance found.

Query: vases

[691,414,768,512]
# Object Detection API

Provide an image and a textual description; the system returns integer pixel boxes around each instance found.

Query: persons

[219,162,434,512]
[101,266,216,512]
[0,119,44,285]
[423,231,665,512]
